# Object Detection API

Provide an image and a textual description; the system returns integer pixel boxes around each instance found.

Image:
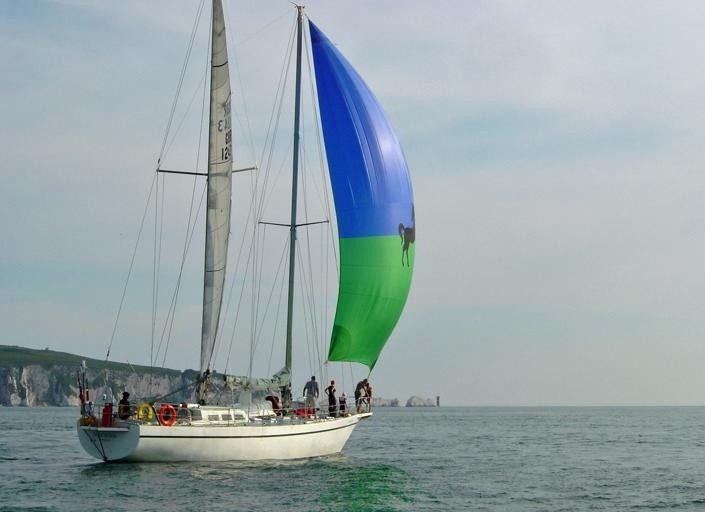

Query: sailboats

[73,2,418,463]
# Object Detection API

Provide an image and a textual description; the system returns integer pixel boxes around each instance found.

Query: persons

[323,380,337,416]
[338,392,350,416]
[118,392,131,421]
[361,382,374,413]
[353,378,370,415]
[302,375,320,419]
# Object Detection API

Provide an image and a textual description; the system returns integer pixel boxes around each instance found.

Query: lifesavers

[159,405,176,425]
[139,404,152,419]
